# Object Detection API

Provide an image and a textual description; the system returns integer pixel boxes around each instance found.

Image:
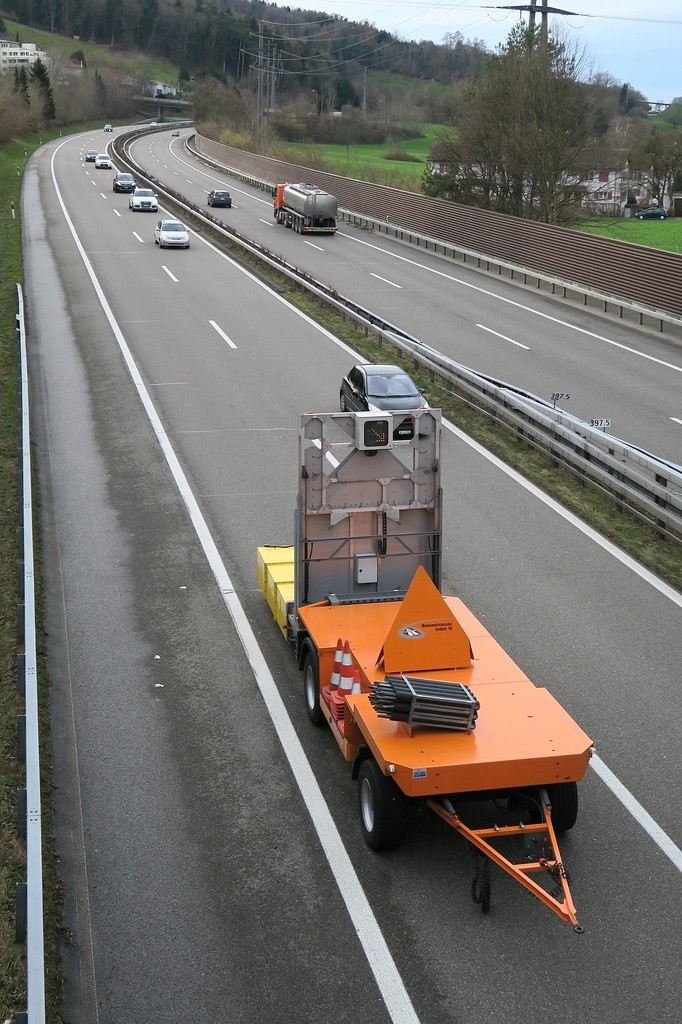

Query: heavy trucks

[271,180,339,236]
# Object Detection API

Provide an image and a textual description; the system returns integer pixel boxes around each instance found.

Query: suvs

[154,218,191,249]
[207,189,232,208]
[129,188,159,212]
[339,363,428,415]
[95,153,113,170]
[113,172,137,193]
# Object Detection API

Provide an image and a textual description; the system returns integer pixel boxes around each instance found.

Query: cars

[85,150,99,162]
[171,129,179,137]
[150,121,158,128]
[635,206,668,220]
[104,124,112,132]
